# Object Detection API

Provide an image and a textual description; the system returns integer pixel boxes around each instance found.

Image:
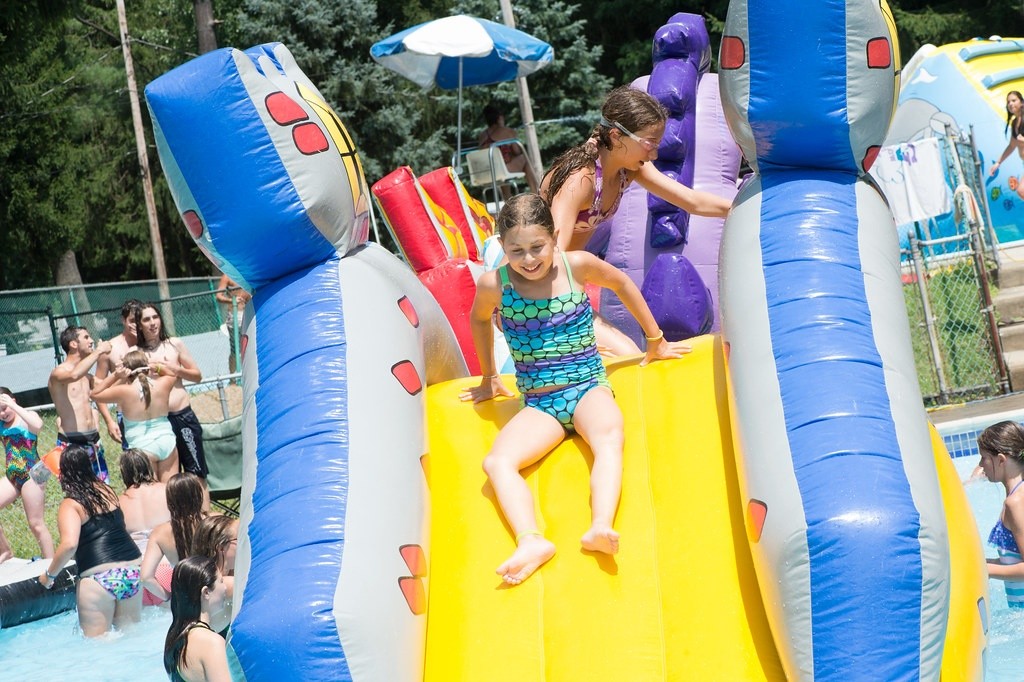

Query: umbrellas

[370,14,554,174]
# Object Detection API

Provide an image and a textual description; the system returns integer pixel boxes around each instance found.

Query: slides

[422,330,786,681]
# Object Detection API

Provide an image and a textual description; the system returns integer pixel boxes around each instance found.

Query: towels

[953,183,985,227]
[867,136,953,227]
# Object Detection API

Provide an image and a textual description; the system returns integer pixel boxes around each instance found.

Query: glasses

[614,121,662,152]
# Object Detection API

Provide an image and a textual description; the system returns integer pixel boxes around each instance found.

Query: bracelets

[156,361,162,374]
[645,330,664,340]
[996,161,1001,164]
[45,568,56,579]
[483,374,498,379]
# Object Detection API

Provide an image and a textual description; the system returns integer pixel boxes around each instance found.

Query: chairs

[466,148,529,213]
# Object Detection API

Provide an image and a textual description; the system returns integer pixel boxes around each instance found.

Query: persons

[38,445,143,637]
[116,448,172,611]
[139,473,238,606]
[162,596,170,602]
[989,91,1024,198]
[163,557,231,682]
[216,273,251,386]
[478,104,537,199]
[493,88,732,355]
[0,386,55,563]
[963,420,1023,610]
[193,516,241,641]
[48,298,210,485]
[459,193,692,585]
